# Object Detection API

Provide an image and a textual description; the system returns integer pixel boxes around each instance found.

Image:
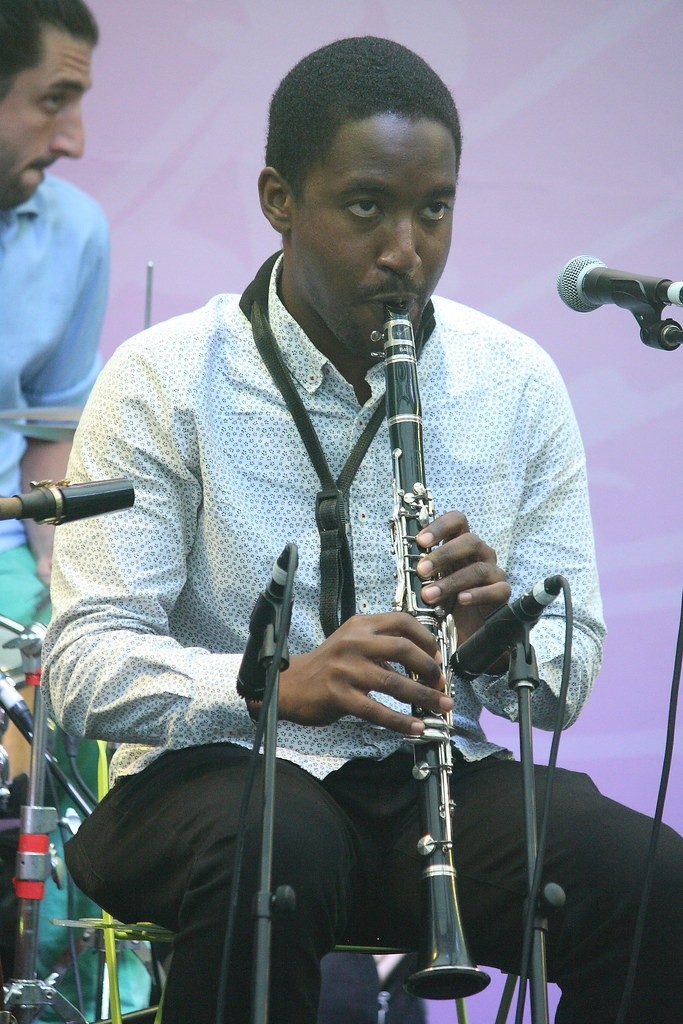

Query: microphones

[449,573,564,683]
[557,253,683,313]
[237,544,298,704]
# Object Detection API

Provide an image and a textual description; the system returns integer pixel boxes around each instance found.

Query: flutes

[383,300,492,999]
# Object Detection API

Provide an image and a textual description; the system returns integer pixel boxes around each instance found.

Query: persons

[0,0,156,1022]
[42,36,683,1024]
